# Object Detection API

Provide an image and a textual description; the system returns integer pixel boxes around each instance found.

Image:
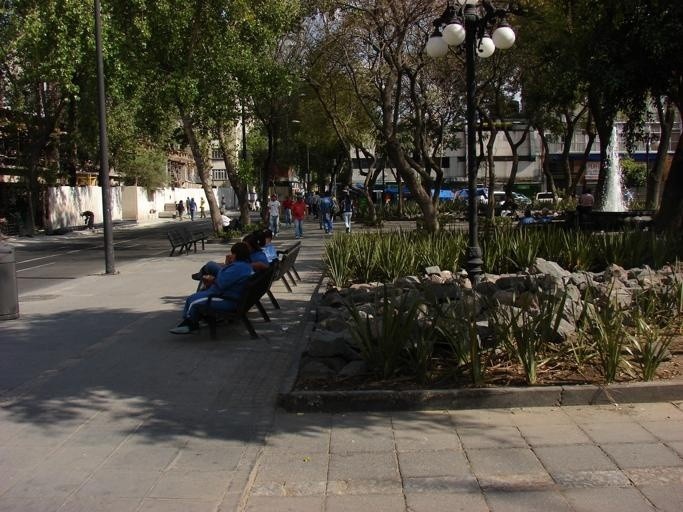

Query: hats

[296,195,304,198]
[270,193,277,197]
[262,228,272,237]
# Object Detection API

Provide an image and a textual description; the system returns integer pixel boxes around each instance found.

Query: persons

[169,187,393,333]
[79,210,94,231]
[496,185,629,225]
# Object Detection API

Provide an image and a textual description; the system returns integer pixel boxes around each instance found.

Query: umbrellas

[385,185,488,200]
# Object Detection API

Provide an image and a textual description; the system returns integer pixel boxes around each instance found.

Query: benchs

[167,223,209,257]
[194,258,280,339]
[270,241,302,292]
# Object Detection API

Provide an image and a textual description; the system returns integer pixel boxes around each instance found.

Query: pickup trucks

[533,191,563,207]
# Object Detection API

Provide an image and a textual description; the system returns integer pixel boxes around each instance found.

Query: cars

[486,191,506,207]
[512,192,531,210]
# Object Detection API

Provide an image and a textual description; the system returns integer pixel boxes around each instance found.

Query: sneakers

[271,232,274,235]
[190,328,199,334]
[301,235,303,238]
[167,327,189,334]
[275,233,278,238]
[320,228,334,236]
[295,235,299,239]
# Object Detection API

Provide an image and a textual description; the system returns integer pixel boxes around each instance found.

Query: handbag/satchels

[329,201,338,216]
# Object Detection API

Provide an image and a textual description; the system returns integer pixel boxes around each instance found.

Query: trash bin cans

[0,244,20,320]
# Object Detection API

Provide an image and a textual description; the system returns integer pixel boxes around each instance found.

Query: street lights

[285,92,305,167]
[291,120,309,194]
[426,0,516,316]
[643,125,651,190]
[374,126,386,220]
